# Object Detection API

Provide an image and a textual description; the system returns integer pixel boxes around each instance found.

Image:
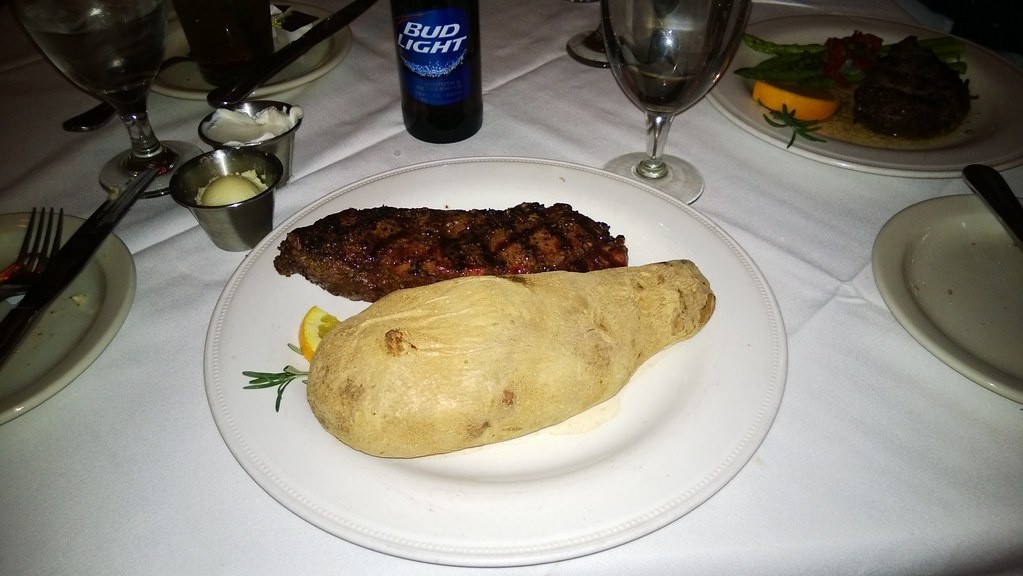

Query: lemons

[299,305,341,363]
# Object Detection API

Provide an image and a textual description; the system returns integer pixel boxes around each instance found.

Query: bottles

[389,1,482,144]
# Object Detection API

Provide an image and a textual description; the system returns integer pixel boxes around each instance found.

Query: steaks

[271,201,631,304]
[855,32,973,140]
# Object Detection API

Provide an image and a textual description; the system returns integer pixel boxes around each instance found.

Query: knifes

[0,167,159,369]
[208,0,379,108]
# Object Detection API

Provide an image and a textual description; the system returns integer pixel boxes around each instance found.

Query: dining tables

[1,1,1023,575]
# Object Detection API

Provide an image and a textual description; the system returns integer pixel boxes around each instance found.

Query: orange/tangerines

[751,78,839,122]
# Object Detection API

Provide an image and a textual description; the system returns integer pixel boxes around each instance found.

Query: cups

[173,0,276,87]
[168,150,283,253]
[198,101,303,189]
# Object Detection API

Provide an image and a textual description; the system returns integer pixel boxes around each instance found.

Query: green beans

[733,30,969,86]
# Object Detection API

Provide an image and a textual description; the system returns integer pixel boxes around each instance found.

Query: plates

[0,207,136,426]
[870,194,1023,404]
[204,157,789,565]
[147,4,353,98]
[705,12,1023,179]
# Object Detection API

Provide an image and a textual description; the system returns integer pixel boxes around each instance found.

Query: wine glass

[9,0,204,197]
[600,0,754,205]
[567,15,610,71]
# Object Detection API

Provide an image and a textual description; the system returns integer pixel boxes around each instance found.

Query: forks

[0,205,65,304]
